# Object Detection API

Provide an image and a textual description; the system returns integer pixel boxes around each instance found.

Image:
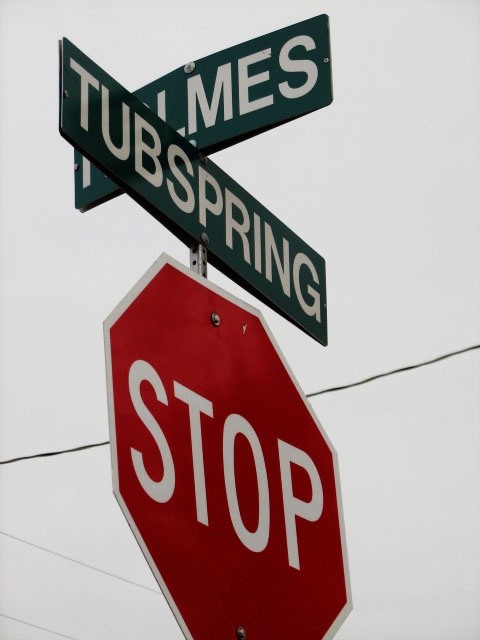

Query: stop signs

[103,253,353,640]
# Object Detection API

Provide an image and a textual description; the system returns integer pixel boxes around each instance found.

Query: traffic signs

[74,14,331,207]
[61,36,326,345]
